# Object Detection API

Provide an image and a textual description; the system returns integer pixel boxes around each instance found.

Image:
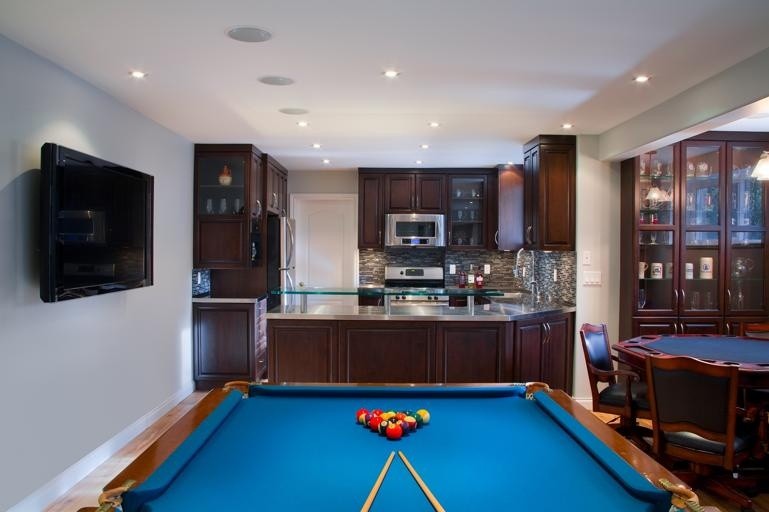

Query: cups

[685,263,693,280]
[638,262,648,279]
[689,290,716,309]
[664,262,673,279]
[699,256,713,280]
[638,289,646,309]
[650,262,662,279]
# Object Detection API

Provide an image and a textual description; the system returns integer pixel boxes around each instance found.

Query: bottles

[467,264,475,289]
[474,270,484,289]
[458,272,466,288]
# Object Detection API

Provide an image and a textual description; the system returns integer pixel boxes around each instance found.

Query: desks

[610,334,769,455]
[76,380,723,512]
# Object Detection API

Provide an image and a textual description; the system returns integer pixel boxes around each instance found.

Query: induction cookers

[384,280,444,294]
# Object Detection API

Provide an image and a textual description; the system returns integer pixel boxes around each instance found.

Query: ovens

[384,287,450,307]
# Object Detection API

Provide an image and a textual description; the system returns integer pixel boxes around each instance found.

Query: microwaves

[384,214,447,249]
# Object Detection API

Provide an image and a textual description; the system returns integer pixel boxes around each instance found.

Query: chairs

[579,322,653,452]
[737,318,769,341]
[643,349,766,512]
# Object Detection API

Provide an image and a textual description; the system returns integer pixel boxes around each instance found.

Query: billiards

[357,407,430,439]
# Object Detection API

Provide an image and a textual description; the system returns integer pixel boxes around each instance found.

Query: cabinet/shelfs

[192,143,267,391]
[473,295,489,305]
[359,295,383,306]
[449,294,468,307]
[382,169,445,213]
[525,135,576,253]
[724,130,768,340]
[262,153,290,215]
[514,312,574,398]
[489,164,525,253]
[446,169,488,252]
[355,168,385,251]
[619,131,723,382]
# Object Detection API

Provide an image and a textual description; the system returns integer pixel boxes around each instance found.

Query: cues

[360,451,396,512]
[399,451,445,512]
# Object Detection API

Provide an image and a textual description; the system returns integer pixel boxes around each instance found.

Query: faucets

[514,246,536,294]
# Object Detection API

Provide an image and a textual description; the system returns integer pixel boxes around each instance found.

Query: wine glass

[639,231,659,245]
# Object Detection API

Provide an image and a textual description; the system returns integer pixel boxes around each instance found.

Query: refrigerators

[266,216,297,311]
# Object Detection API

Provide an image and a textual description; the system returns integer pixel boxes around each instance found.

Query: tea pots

[727,285,745,309]
[730,255,754,278]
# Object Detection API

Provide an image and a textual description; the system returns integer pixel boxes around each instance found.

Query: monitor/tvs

[41,141,155,303]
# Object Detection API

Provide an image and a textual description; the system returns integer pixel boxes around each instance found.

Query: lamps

[646,152,671,204]
[750,148,769,182]
